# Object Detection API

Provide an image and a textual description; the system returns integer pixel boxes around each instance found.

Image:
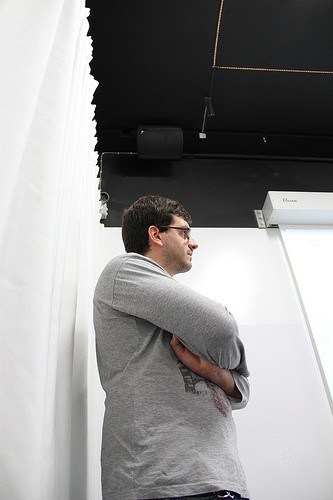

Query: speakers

[137,126,183,159]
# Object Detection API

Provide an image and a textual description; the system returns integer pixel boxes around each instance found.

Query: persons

[92,195,251,500]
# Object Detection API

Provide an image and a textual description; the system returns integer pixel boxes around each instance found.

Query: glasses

[157,225,191,240]
